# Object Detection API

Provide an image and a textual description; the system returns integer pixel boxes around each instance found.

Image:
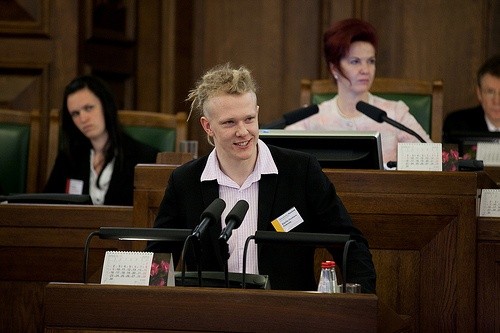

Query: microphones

[218,200,249,242]
[263,105,320,129]
[105,126,125,164]
[192,198,226,239]
[356,101,427,144]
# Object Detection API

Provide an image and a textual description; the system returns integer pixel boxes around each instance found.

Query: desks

[0,164,500,333]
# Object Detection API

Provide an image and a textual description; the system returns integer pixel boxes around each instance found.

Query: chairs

[0,108,41,191]
[301,77,444,144]
[45,109,187,198]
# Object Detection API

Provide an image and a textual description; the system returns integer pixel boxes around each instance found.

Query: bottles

[326,261,338,293]
[317,262,334,293]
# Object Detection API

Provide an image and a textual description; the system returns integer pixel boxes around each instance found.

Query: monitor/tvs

[260,133,385,169]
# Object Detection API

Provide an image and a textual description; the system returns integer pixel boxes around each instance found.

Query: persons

[441,58,500,144]
[144,62,376,294]
[42,75,160,206]
[285,20,434,170]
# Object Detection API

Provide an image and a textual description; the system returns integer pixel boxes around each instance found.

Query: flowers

[149,258,169,286]
[442,150,458,171]
[463,146,476,160]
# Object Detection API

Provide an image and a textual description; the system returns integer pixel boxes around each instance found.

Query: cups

[179,141,198,160]
[338,283,362,293]
